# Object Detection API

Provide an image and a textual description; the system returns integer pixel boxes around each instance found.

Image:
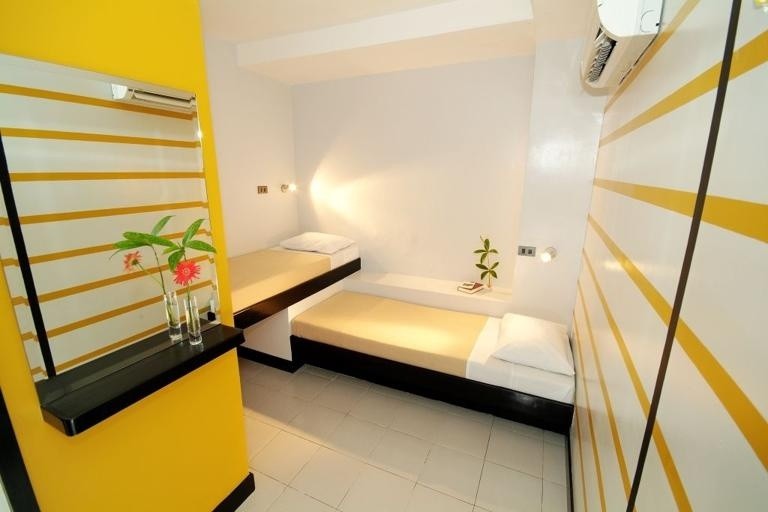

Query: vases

[185,296,203,346]
[163,290,182,329]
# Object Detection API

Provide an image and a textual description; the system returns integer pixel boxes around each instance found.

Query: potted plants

[472,233,500,290]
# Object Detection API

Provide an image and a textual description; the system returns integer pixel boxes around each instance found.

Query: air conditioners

[112,83,196,115]
[575,0,663,99]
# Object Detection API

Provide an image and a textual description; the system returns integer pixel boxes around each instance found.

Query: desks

[227,240,362,374]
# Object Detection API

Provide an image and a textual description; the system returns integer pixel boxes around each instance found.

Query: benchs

[291,290,577,438]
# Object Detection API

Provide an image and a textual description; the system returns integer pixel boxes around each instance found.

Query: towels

[280,229,356,256]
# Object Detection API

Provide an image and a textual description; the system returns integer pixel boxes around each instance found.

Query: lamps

[539,246,557,262]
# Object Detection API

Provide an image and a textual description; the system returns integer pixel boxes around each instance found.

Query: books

[457,282,484,294]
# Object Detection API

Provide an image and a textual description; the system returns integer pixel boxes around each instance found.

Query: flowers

[122,219,218,333]
[108,213,185,326]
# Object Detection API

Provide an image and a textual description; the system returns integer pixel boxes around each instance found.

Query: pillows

[491,310,576,377]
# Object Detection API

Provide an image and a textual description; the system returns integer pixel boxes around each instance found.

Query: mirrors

[2,53,221,406]
